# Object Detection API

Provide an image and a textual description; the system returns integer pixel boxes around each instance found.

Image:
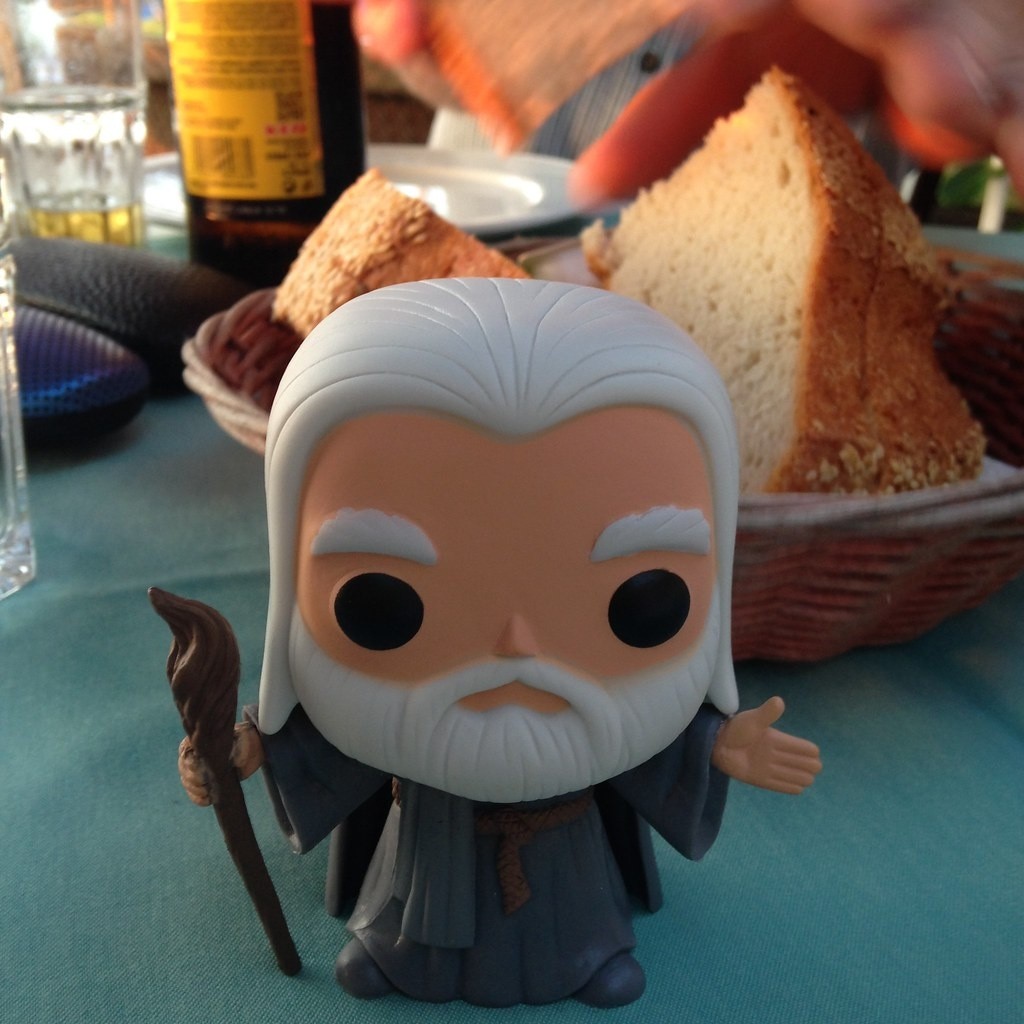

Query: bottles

[159,0,367,276]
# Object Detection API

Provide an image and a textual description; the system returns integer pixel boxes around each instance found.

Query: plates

[139,139,578,236]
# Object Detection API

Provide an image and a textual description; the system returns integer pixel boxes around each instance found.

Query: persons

[364,0,1024,218]
[181,276,820,1009]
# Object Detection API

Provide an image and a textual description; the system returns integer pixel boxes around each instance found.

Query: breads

[273,166,529,337]
[578,64,986,493]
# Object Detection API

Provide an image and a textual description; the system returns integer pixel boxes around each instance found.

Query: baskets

[178,237,1024,662]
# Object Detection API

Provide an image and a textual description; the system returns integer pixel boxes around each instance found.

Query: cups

[0,0,153,252]
